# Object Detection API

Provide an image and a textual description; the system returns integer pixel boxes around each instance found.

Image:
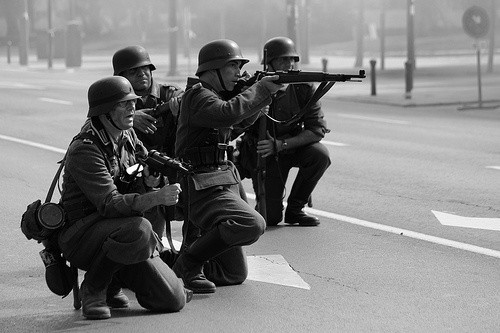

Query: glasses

[118,99,138,107]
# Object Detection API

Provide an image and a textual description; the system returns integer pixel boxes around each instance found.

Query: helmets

[87,76,142,117]
[112,46,157,76]
[195,39,249,75]
[261,37,299,64]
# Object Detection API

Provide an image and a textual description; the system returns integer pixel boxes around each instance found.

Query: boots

[78,253,129,319]
[284,170,321,226]
[171,226,232,294]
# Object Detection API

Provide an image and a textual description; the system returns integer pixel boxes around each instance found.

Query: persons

[113,45,185,240]
[172,38,284,293]
[236,37,332,226]
[60,76,186,319]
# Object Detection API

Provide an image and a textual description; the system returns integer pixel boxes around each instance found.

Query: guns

[235,70,366,85]
[137,149,195,179]
[145,97,181,123]
[256,50,268,232]
